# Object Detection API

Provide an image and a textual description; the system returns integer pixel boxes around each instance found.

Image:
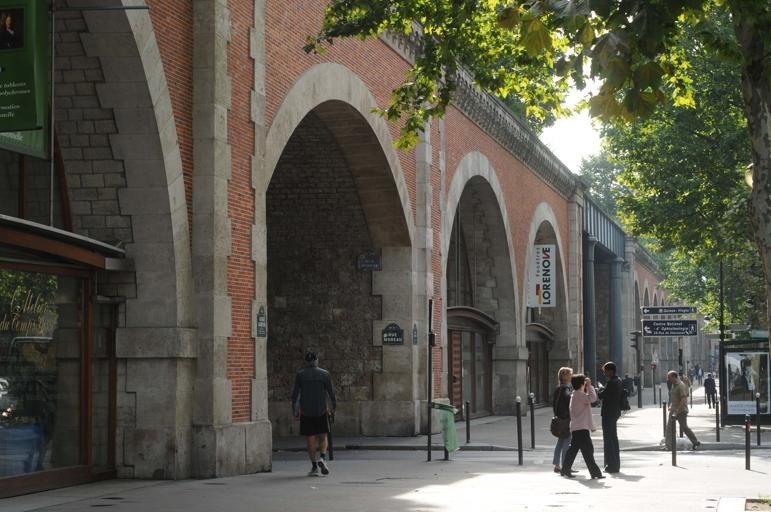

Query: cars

[0,336,56,419]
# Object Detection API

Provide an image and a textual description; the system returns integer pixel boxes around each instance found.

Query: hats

[305,352,317,361]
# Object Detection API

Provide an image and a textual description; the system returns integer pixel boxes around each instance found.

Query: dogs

[659,437,694,451]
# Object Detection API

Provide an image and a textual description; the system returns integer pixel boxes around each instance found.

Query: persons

[563,373,608,481]
[659,370,701,453]
[703,373,717,409]
[620,373,635,396]
[547,366,573,475]
[678,365,703,387]
[290,351,337,478]
[598,362,629,474]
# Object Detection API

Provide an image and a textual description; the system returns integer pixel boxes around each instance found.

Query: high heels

[554,467,579,478]
[592,475,605,478]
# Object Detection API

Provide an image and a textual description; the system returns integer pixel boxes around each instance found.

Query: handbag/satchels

[620,389,630,410]
[551,416,561,436]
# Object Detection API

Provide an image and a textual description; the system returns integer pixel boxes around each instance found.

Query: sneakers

[690,442,700,450]
[308,460,329,476]
